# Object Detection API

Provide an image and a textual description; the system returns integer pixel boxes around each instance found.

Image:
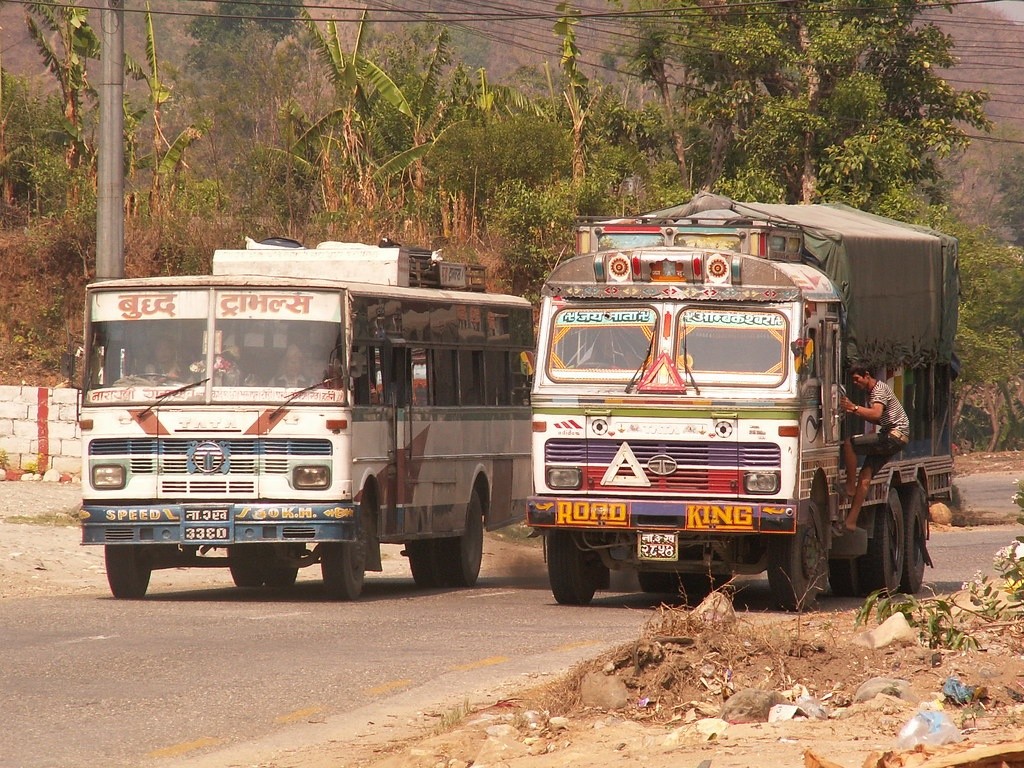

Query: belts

[889,429,910,444]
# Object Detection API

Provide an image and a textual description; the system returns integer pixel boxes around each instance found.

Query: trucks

[520,191,953,613]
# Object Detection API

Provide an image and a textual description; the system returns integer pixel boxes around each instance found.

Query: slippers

[834,483,854,500]
[834,521,854,534]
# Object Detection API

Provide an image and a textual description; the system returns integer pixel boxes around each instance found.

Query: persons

[835,365,911,532]
[123,335,201,386]
[265,340,320,388]
[320,356,378,405]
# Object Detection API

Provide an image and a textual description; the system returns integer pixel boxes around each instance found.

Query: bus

[61,248,536,602]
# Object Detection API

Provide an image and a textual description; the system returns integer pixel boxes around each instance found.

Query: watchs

[853,405,859,412]
